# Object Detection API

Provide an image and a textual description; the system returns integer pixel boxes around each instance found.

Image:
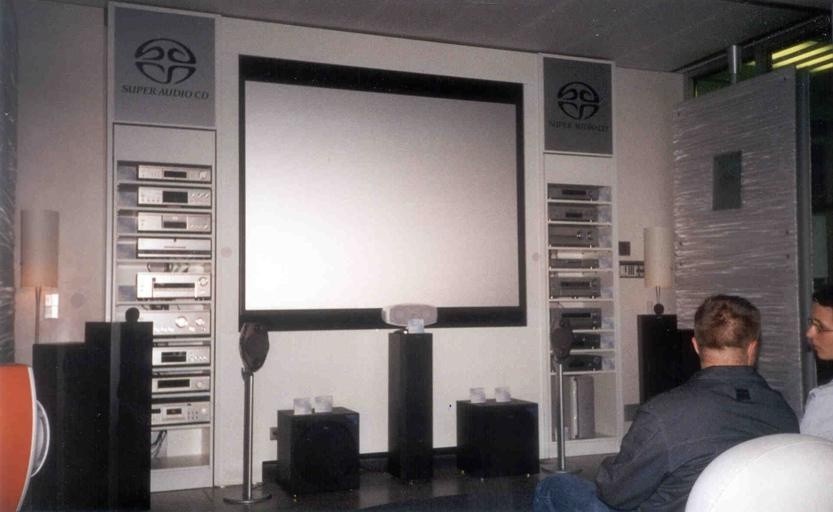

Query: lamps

[643,225,674,316]
[20,209,57,345]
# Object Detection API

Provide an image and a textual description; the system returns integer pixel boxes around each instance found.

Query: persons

[533,294,801,511]
[802,276,833,440]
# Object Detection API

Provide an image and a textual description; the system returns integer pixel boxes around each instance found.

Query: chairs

[685,434,833,512]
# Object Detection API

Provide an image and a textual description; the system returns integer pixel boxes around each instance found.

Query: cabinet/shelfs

[112,122,215,493]
[543,150,622,460]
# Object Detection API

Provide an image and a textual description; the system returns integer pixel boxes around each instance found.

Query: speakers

[238,320,271,370]
[387,333,433,478]
[84,320,154,512]
[382,303,437,334]
[549,317,573,360]
[32,343,84,512]
[636,314,678,407]
[457,399,540,479]
[677,329,700,384]
[276,408,361,496]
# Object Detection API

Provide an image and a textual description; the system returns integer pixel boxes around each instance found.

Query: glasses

[808,318,833,333]
